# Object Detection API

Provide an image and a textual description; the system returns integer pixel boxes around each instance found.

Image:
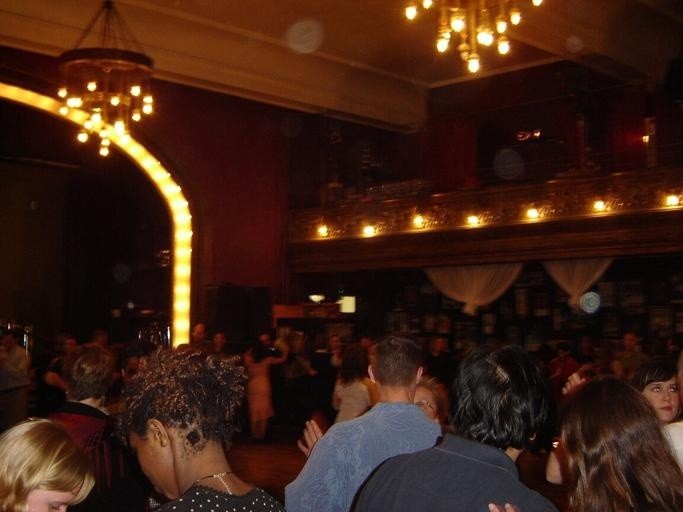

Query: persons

[0,305,683,511]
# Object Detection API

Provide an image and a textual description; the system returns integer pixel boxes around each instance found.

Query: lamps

[404,0,546,76]
[50,0,156,158]
